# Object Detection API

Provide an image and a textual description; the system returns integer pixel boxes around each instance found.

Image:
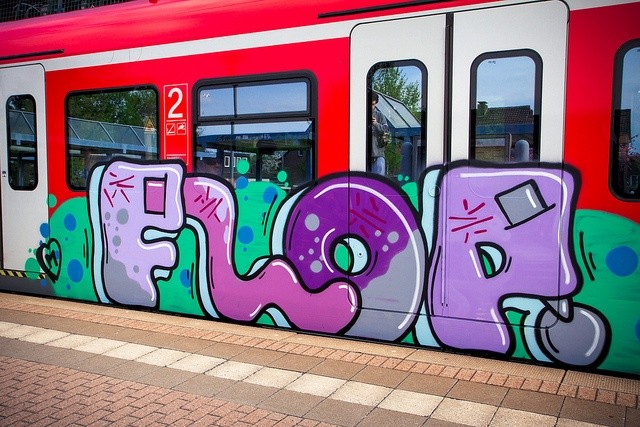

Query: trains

[1,0,640,379]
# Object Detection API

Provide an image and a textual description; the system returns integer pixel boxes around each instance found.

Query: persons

[370,92,390,175]
